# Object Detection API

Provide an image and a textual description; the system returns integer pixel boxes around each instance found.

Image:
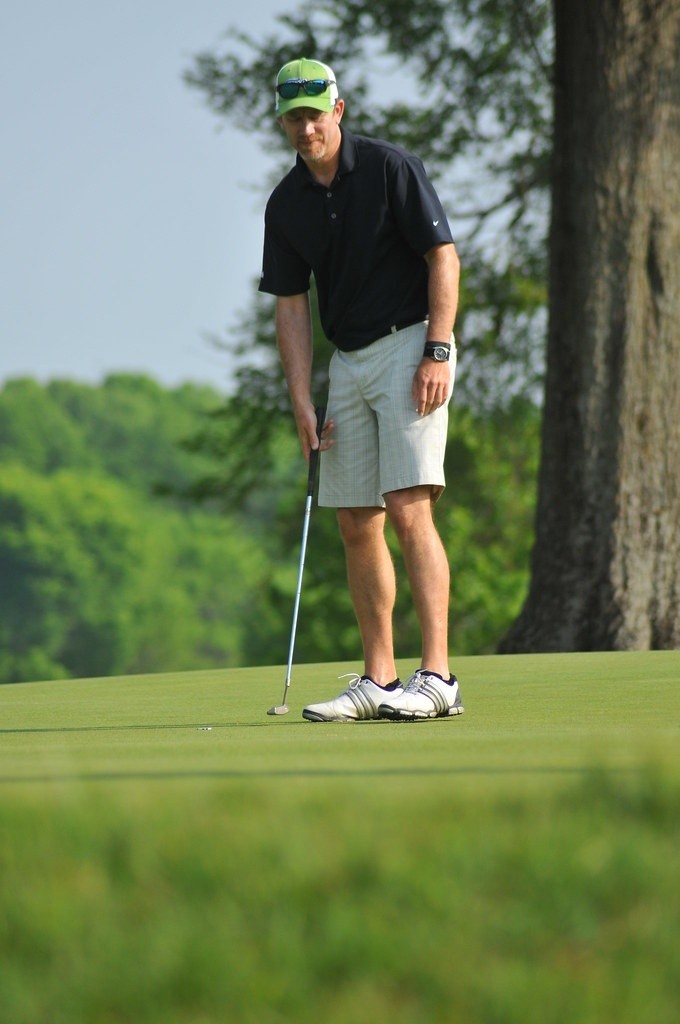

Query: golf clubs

[267,405,325,716]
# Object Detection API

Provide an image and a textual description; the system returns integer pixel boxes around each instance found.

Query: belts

[336,312,431,353]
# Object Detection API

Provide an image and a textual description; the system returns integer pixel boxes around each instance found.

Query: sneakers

[301,674,405,722]
[378,668,465,719]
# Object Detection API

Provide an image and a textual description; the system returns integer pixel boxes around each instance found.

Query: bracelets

[425,342,451,351]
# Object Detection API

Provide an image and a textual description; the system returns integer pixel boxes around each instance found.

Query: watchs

[423,346,450,362]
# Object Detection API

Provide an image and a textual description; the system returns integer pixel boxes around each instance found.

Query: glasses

[275,79,336,100]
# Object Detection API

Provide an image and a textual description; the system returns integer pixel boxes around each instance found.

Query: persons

[258,57,465,722]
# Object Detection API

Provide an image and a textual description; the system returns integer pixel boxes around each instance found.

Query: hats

[274,58,339,116]
